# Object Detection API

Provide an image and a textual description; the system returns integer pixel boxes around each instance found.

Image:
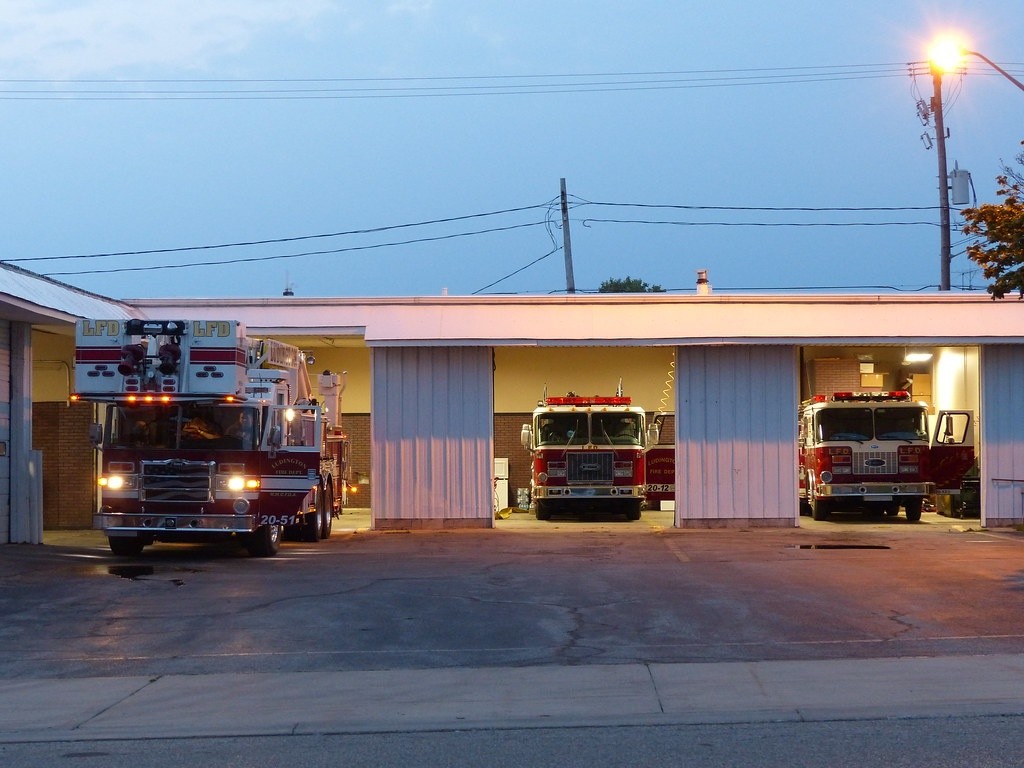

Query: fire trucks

[799,391,975,521]
[521,376,675,520]
[75,318,347,554]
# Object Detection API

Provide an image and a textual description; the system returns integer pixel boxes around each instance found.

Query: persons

[225,410,259,446]
[127,420,157,449]
